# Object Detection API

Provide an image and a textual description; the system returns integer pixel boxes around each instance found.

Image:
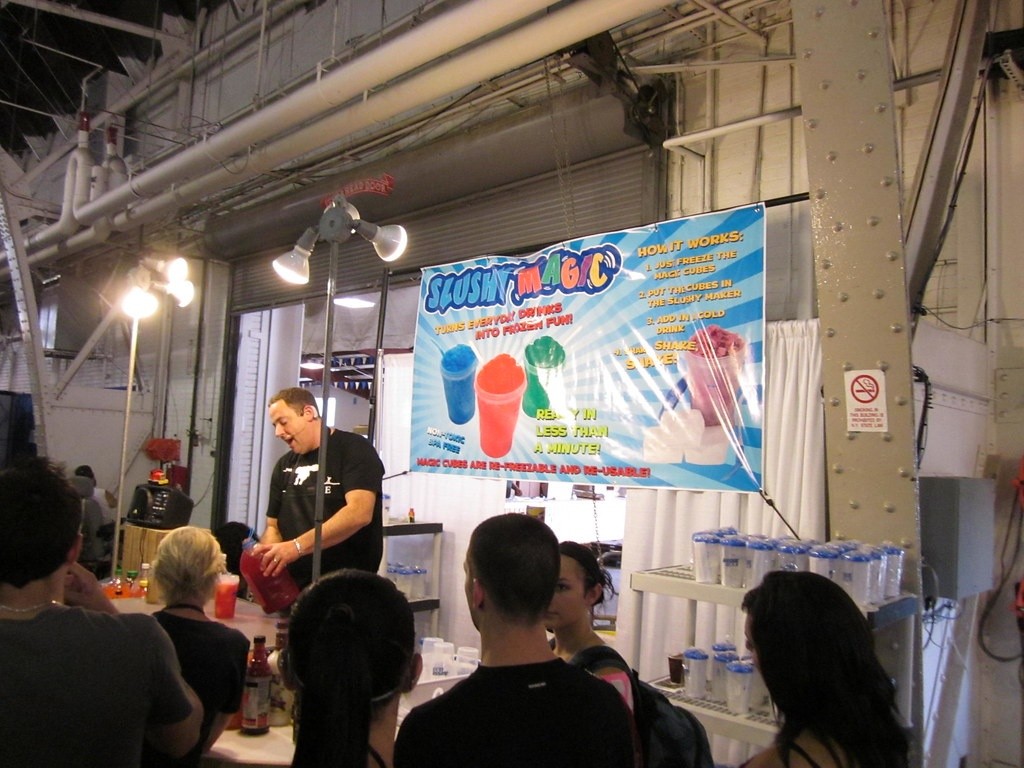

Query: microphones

[287,426,305,439]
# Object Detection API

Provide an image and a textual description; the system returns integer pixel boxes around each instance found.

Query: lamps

[109,257,195,575]
[273,190,409,583]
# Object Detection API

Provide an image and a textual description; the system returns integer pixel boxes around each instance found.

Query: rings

[273,558,278,564]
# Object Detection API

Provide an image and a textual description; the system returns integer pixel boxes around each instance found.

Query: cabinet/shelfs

[627,565,917,748]
[377,517,443,638]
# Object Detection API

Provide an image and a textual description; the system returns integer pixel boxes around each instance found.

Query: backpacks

[547,636,714,768]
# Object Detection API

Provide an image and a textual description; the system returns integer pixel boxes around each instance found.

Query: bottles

[240,609,295,733]
[101,561,155,602]
[240,526,300,613]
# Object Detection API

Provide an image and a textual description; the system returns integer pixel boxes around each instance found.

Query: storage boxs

[398,653,481,717]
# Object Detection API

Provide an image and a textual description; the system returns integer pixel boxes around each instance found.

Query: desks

[104,595,296,768]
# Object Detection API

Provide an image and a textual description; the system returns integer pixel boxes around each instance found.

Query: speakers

[125,484,194,530]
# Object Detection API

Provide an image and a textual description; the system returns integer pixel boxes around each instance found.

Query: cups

[215,575,240,620]
[474,353,528,458]
[693,526,906,608]
[521,335,566,419]
[686,332,746,432]
[415,636,478,683]
[386,562,428,602]
[440,345,478,423]
[667,640,786,722]
[382,495,389,525]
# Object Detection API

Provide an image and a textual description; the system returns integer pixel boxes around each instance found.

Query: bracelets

[293,538,304,557]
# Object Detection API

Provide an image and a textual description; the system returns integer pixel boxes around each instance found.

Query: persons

[250,386,385,592]
[74,464,118,540]
[735,570,916,768]
[138,525,251,768]
[276,513,643,768]
[0,455,205,768]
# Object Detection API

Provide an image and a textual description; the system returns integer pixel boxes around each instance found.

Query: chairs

[69,476,112,561]
